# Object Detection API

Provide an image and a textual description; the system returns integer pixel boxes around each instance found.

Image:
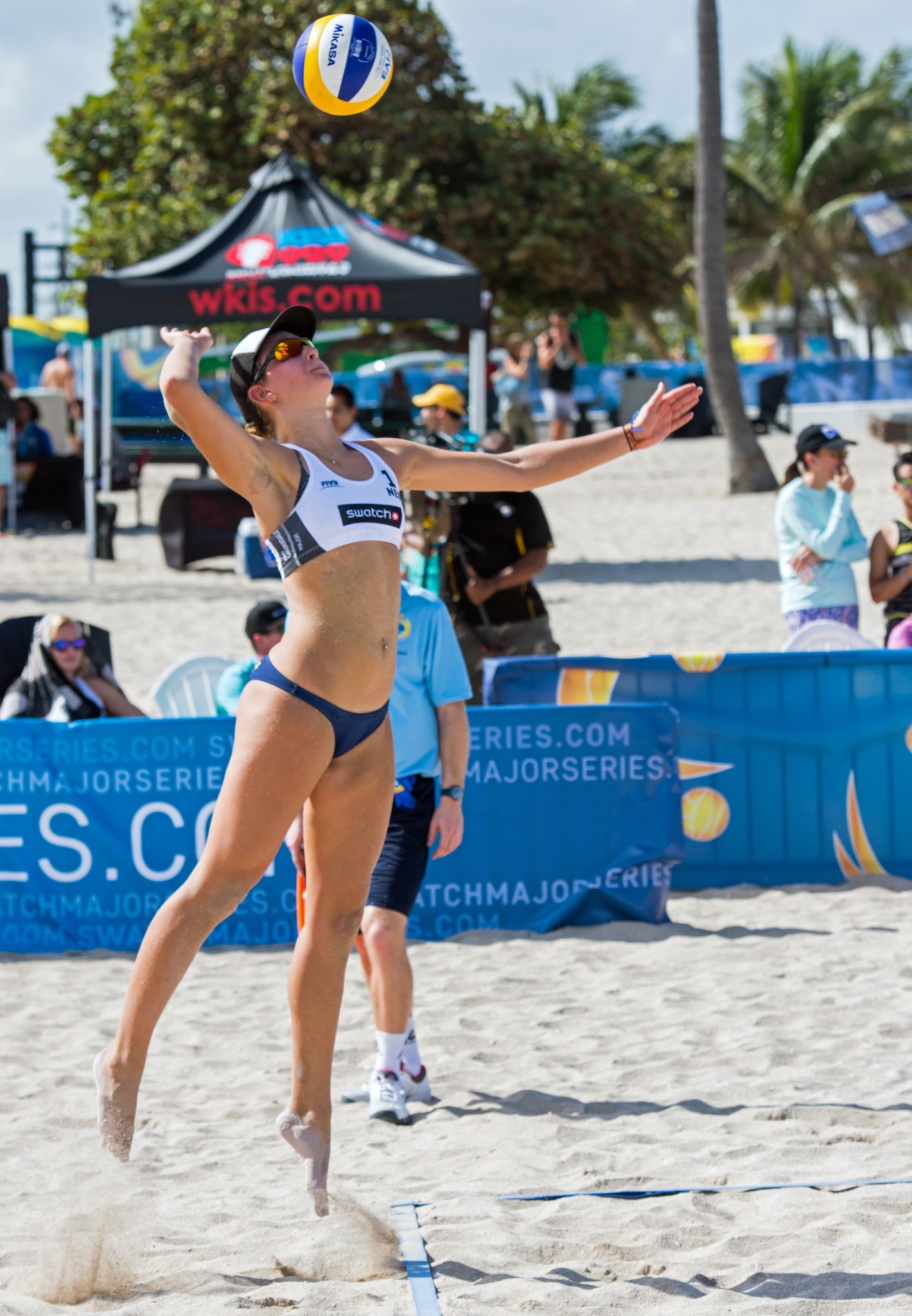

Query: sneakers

[370,1073,412,1126]
[341,1064,432,1104]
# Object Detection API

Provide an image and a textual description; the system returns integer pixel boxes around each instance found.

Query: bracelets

[622,411,658,453]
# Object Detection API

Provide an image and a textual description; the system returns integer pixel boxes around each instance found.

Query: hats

[411,383,465,416]
[245,602,288,638]
[796,424,857,456]
[229,305,317,424]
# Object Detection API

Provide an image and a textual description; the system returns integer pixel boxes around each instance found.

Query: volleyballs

[292,13,394,116]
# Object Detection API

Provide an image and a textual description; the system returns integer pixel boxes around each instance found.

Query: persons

[778,423,871,639]
[282,579,474,1129]
[0,304,640,552]
[868,451,912,651]
[0,613,151,722]
[210,601,289,717]
[432,431,563,706]
[760,370,793,437]
[97,301,701,1217]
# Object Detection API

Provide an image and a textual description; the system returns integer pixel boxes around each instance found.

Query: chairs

[0,616,113,705]
[151,654,233,719]
[757,369,792,435]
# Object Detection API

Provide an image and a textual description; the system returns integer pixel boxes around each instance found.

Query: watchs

[440,784,465,801]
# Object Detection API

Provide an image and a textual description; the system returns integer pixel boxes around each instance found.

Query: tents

[85,150,482,558]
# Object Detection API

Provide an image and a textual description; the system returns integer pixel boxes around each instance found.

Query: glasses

[245,337,315,404]
[898,476,912,487]
[813,450,847,459]
[51,637,88,651]
